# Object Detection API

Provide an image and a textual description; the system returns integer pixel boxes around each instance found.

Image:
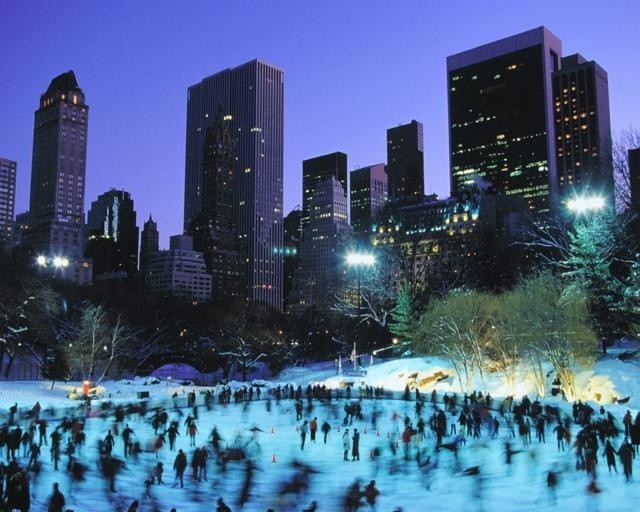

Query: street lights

[344,251,378,359]
